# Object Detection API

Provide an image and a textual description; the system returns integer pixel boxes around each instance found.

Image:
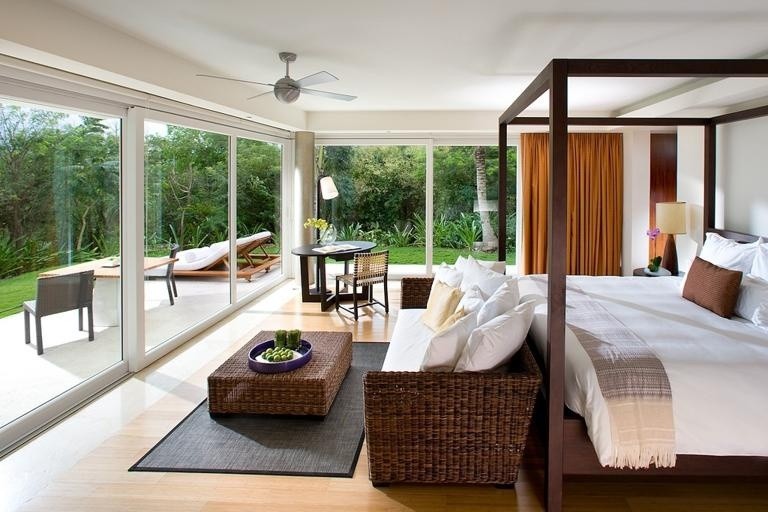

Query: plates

[248,338,313,374]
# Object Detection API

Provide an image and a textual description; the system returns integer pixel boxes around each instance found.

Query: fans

[196,52,358,102]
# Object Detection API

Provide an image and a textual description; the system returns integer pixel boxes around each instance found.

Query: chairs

[162,231,280,282]
[23,270,95,355]
[144,244,179,305]
[335,250,389,321]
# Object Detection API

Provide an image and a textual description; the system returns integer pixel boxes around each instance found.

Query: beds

[498,59,768,512]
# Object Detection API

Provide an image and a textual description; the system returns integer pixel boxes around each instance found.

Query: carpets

[127,342,390,478]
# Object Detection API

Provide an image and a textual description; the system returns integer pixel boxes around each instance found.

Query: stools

[208,331,352,423]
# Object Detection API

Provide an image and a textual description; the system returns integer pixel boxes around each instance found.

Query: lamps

[656,202,686,276]
[317,176,339,244]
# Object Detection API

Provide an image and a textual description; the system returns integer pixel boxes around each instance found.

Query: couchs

[362,275,543,489]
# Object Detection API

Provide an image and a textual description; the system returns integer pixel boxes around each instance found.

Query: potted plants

[304,217,337,245]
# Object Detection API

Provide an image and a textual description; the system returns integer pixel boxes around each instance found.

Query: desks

[290,241,377,302]
[38,256,179,327]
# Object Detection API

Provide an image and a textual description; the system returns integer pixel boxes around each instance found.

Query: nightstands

[633,267,685,278]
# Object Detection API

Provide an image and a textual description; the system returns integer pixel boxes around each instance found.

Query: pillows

[751,243,768,281]
[734,272,768,326]
[682,256,743,320]
[419,311,477,373]
[475,259,506,274]
[699,232,764,274]
[454,284,484,315]
[459,255,512,302]
[427,261,463,310]
[477,276,521,326]
[436,306,465,334]
[452,255,496,277]
[420,279,462,331]
[453,299,537,373]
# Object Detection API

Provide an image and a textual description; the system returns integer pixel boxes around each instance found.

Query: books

[312,244,362,254]
